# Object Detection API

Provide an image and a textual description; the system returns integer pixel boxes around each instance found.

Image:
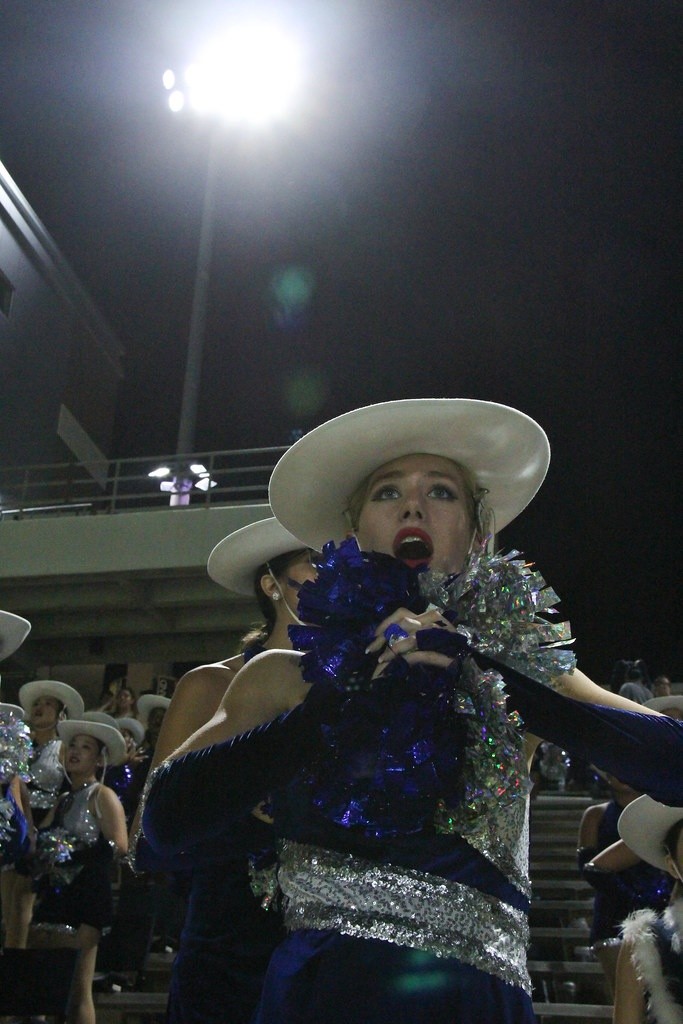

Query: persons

[125,517,318,1024]
[0,609,170,1024]
[525,656,683,1023]
[141,397,683,1024]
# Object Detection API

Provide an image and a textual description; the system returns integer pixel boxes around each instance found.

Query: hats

[1,682,171,769]
[269,399,552,553]
[1,609,31,659]
[643,695,682,718]
[614,792,683,872]
[204,519,305,594]
